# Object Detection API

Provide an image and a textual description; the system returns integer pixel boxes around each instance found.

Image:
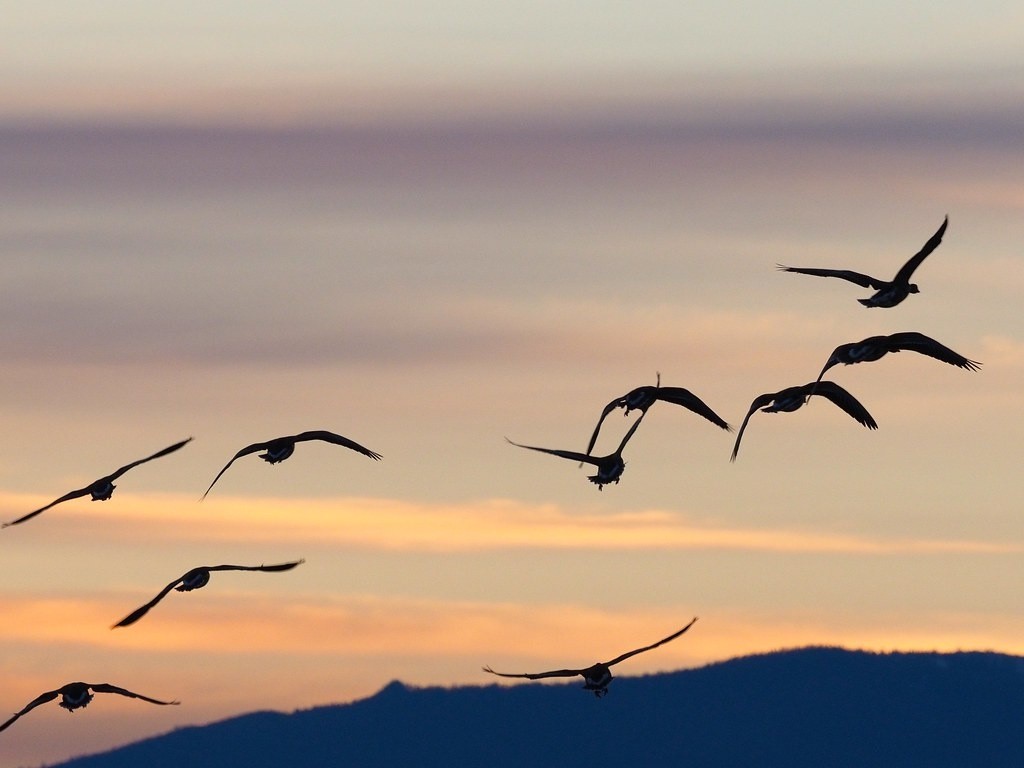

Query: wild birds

[112,560,305,627]
[774,213,949,308]
[482,618,699,697]
[729,382,879,462]
[0,683,182,736]
[3,437,200,530]
[581,386,737,468]
[503,413,646,491]
[201,431,383,502]
[806,333,984,407]
